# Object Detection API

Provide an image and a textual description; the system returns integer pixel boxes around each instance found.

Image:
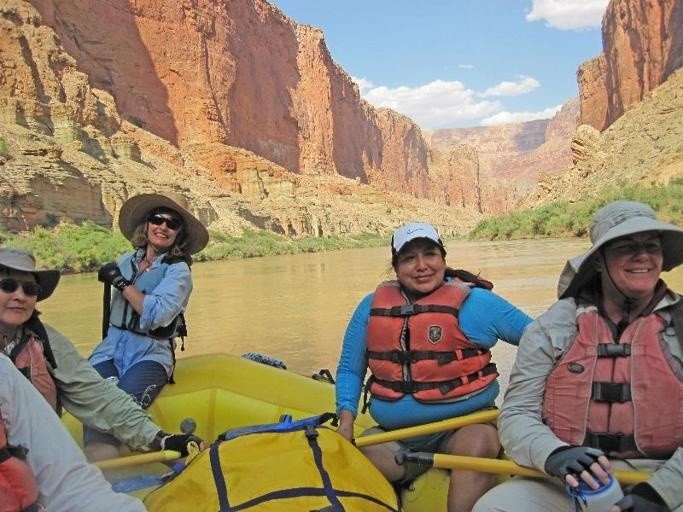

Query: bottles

[566,472,624,511]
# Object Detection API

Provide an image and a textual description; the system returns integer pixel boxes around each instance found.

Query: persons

[0,248,205,466]
[0,353,148,512]
[472,200,683,512]
[82,192,209,486]
[335,222,535,512]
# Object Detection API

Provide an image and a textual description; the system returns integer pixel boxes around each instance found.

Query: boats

[58,347,517,512]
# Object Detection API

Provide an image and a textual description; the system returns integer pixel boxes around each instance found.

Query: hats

[390,221,447,267]
[119,191,209,256]
[558,199,683,300]
[0,248,60,302]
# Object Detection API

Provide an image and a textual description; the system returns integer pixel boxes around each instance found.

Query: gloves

[98,260,132,292]
[545,444,605,484]
[163,431,203,457]
[617,488,670,511]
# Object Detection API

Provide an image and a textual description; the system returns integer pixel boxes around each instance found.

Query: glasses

[0,278,42,295]
[146,213,181,231]
